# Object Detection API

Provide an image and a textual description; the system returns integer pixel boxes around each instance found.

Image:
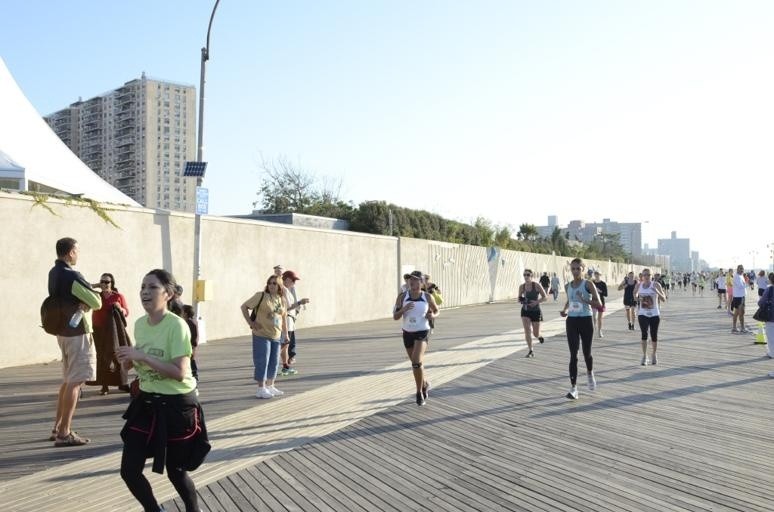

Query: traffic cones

[753,322,767,344]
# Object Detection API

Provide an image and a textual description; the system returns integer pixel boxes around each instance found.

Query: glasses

[523,274,532,277]
[99,279,112,285]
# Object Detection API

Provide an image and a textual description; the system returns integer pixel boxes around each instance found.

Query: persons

[559,259,603,399]
[395,271,444,408]
[242,265,312,400]
[519,237,774,368]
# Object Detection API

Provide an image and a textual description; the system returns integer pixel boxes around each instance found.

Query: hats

[404,270,425,281]
[281,271,300,281]
[273,265,284,269]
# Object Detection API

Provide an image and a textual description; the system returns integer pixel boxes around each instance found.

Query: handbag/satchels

[39,295,57,336]
[250,308,257,329]
[752,303,770,322]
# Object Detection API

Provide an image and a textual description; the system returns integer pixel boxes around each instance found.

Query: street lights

[749,250,760,272]
[766,242,774,273]
[630,218,652,277]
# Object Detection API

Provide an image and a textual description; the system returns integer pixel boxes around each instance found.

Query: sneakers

[566,388,580,400]
[98,386,111,396]
[586,371,597,391]
[628,322,635,331]
[731,326,754,335]
[118,384,131,393]
[282,367,297,376]
[525,351,536,358]
[641,356,650,366]
[416,391,425,406]
[288,356,296,364]
[538,335,544,344]
[265,383,284,396]
[49,427,91,447]
[422,380,431,400]
[598,330,604,339]
[651,353,657,365]
[255,387,274,399]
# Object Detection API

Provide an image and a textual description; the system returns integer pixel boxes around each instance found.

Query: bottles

[303,301,307,311]
[68,307,86,329]
